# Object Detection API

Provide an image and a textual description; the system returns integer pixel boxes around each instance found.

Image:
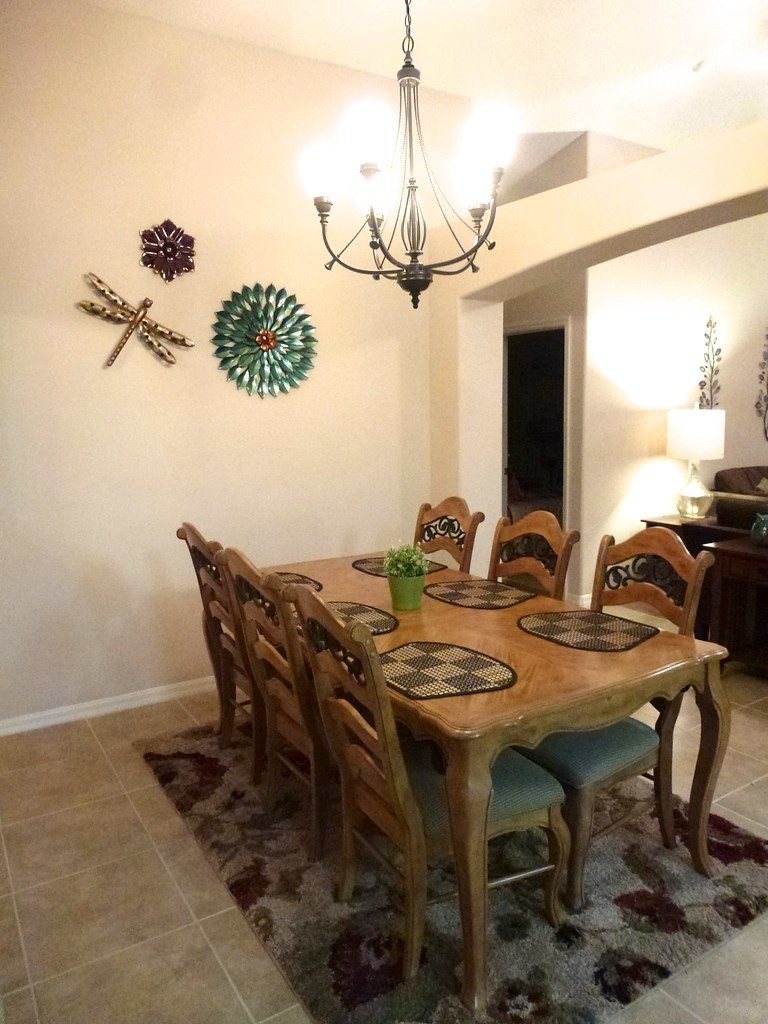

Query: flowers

[382,538,430,579]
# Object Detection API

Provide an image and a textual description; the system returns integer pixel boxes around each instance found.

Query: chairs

[176,521,568,990]
[511,525,715,909]
[414,497,486,574]
[487,511,582,599]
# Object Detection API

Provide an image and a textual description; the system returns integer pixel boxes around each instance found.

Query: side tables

[639,514,717,604]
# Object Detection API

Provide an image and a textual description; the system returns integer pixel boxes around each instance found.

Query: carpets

[131,714,768,1024]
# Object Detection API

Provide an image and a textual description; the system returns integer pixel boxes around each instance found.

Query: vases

[387,575,425,611]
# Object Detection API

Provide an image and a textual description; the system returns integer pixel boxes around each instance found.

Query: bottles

[751,513,768,546]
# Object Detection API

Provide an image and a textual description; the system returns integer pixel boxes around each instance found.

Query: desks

[701,538,768,676]
[243,551,732,1014]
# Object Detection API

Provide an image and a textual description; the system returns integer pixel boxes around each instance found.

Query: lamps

[299,0,512,309]
[665,402,726,520]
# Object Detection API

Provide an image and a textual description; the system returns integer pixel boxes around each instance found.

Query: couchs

[710,465,768,526]
